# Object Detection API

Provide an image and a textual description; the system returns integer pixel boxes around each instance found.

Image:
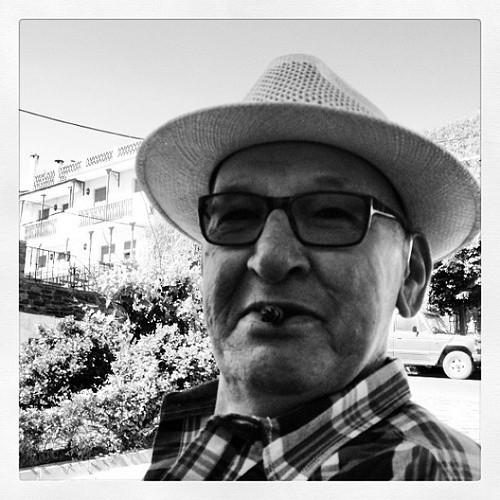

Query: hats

[135,53,482,264]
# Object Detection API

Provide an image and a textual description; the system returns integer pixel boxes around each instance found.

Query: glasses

[196,186,410,250]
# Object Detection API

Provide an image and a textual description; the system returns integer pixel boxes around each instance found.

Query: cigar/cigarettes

[259,305,287,323]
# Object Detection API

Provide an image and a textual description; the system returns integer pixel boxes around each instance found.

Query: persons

[134,52,482,482]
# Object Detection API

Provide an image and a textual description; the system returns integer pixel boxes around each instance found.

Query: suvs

[386,306,478,379]
